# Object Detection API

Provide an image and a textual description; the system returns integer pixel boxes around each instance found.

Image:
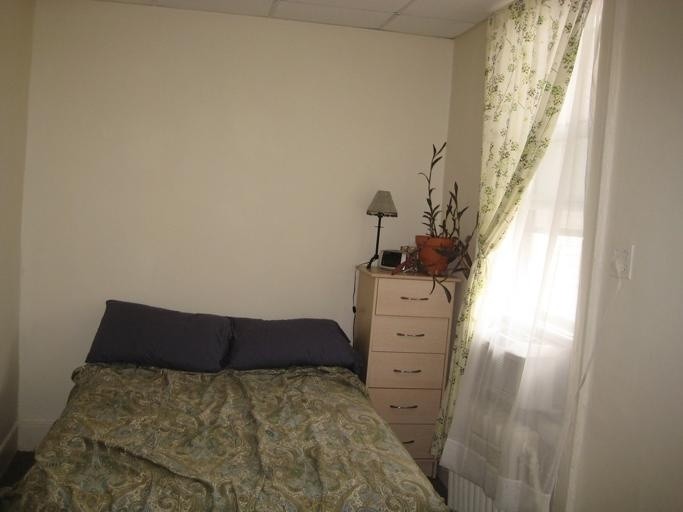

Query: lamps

[364,191,399,269]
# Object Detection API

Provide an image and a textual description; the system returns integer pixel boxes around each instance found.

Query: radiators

[437,401,531,512]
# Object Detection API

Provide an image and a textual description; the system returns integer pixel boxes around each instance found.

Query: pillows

[227,316,363,368]
[85,299,231,372]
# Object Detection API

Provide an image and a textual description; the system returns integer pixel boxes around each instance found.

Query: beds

[0,363,449,511]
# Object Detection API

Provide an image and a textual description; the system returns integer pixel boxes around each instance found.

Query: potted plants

[414,141,470,276]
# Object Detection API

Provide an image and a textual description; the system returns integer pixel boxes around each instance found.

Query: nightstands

[352,264,462,479]
